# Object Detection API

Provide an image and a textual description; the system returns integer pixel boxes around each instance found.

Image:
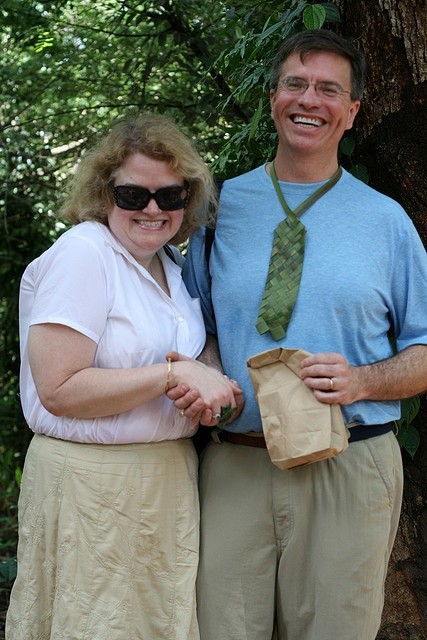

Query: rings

[213,413,221,420]
[177,410,185,417]
[328,377,334,390]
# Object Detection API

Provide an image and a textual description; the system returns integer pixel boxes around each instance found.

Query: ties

[256,160,341,342]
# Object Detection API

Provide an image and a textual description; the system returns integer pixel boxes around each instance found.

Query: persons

[4,113,243,640]
[166,29,427,640]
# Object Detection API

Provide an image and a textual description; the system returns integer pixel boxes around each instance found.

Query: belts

[202,422,395,450]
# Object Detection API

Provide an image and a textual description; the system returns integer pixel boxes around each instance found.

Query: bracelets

[165,358,171,391]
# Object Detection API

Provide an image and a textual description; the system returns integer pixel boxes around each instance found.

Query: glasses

[277,75,354,98]
[109,186,194,210]
